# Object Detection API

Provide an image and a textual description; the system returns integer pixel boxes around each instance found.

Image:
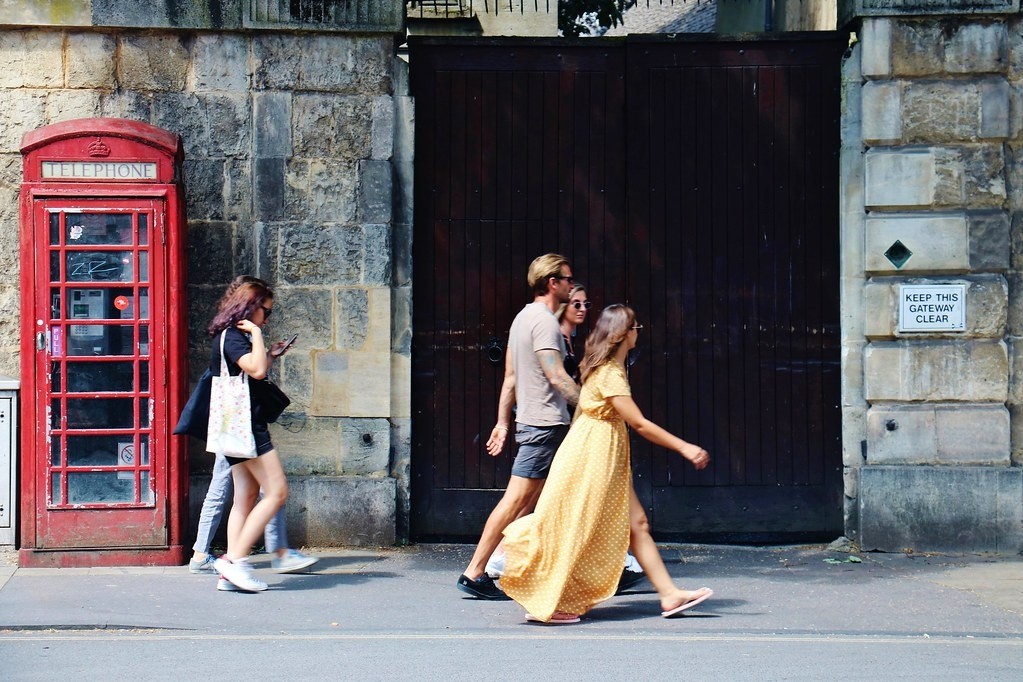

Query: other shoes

[615,568,649,592]
[457,572,511,601]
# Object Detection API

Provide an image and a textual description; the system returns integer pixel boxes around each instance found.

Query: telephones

[53,288,107,342]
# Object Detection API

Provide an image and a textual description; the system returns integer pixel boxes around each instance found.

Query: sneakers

[189,555,220,575]
[271,550,319,574]
[213,554,269,592]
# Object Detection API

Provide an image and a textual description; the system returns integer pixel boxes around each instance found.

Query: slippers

[526,612,580,624]
[661,586,713,619]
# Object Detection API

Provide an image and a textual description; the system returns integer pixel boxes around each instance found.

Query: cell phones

[276,334,298,355]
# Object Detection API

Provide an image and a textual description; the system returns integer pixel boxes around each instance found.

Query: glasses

[568,302,592,310]
[260,305,273,320]
[632,324,643,331]
[556,276,576,284]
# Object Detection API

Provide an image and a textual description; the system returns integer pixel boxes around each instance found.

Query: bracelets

[249,324,258,333]
[494,425,510,432]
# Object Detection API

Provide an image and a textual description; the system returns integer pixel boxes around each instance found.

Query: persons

[493,303,712,624]
[187,274,319,592]
[456,252,646,601]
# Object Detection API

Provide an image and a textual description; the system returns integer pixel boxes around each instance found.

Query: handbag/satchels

[173,367,290,441]
[206,326,259,459]
